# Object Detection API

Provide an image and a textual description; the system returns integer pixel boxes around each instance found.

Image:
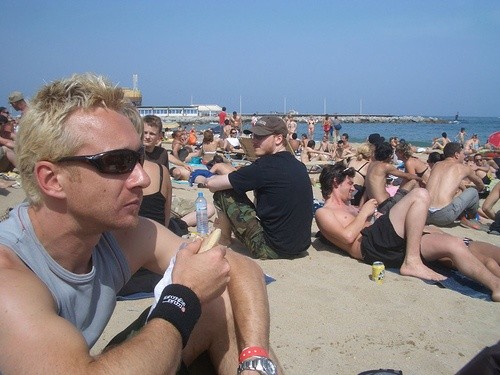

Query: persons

[0,73,284,375]
[0,92,27,188]
[138,107,500,302]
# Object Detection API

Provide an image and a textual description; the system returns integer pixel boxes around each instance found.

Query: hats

[243,116,287,137]
[8,92,23,103]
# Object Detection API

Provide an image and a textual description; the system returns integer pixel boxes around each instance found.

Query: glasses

[232,132,237,134]
[250,134,263,140]
[4,112,9,114]
[59,146,144,175]
[476,159,482,162]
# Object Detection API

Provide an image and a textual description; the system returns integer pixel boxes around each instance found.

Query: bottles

[194,192,208,234]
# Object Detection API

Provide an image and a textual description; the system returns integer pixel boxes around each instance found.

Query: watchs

[237,357,277,375]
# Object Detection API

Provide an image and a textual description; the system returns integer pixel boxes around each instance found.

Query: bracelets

[238,346,269,363]
[147,284,202,349]
